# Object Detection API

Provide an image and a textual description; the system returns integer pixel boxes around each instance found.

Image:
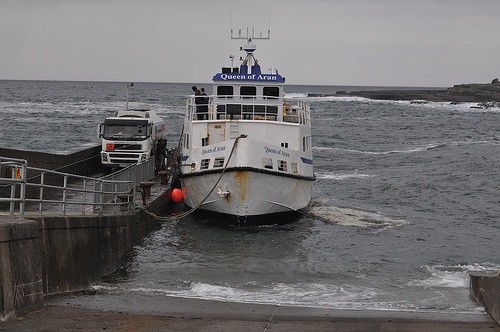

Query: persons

[155,138,163,173]
[192,85,204,120]
[200,88,209,120]
[162,139,168,157]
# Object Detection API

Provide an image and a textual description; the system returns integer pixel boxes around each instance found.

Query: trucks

[98,110,166,176]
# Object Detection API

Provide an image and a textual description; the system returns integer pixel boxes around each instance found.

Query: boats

[179,27,315,228]
